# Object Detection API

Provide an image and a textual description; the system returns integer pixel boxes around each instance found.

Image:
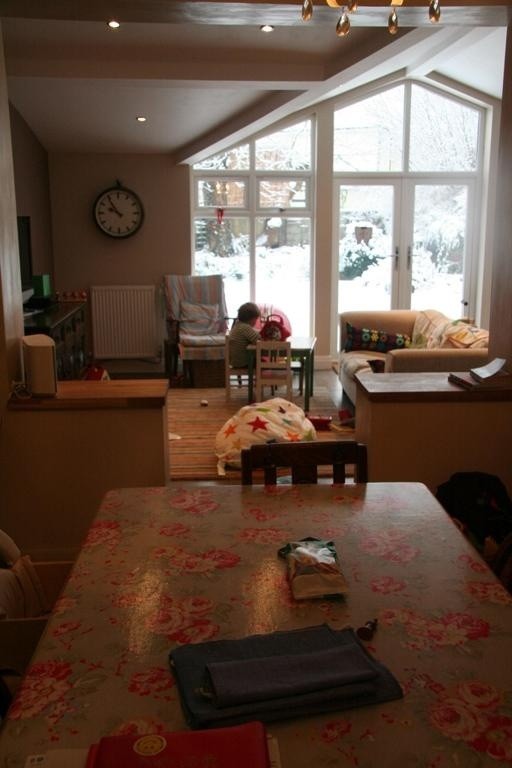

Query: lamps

[301,0,442,37]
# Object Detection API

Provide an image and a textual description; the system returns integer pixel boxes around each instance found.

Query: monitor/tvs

[16,214,35,292]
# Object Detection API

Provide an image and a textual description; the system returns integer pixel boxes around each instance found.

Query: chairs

[225,329,256,400]
[241,441,368,485]
[256,339,293,404]
[270,355,304,397]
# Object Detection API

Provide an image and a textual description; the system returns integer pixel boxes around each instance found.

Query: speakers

[22,333,57,396]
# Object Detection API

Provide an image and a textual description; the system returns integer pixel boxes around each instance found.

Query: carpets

[167,388,356,480]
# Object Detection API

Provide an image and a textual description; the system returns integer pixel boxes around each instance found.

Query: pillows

[367,360,385,373]
[345,322,411,353]
[179,300,219,336]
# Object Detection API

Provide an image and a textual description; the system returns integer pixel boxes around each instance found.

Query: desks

[247,337,317,412]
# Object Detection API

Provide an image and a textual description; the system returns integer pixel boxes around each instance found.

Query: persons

[228,304,265,368]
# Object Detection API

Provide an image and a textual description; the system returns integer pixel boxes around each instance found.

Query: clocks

[92,186,146,240]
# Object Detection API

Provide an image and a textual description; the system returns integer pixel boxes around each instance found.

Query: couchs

[162,275,227,385]
[339,310,489,406]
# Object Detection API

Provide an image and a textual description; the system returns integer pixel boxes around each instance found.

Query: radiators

[90,285,158,359]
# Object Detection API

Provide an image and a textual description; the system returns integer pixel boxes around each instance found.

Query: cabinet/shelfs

[23,301,88,381]
[7,380,169,569]
[353,373,512,493]
